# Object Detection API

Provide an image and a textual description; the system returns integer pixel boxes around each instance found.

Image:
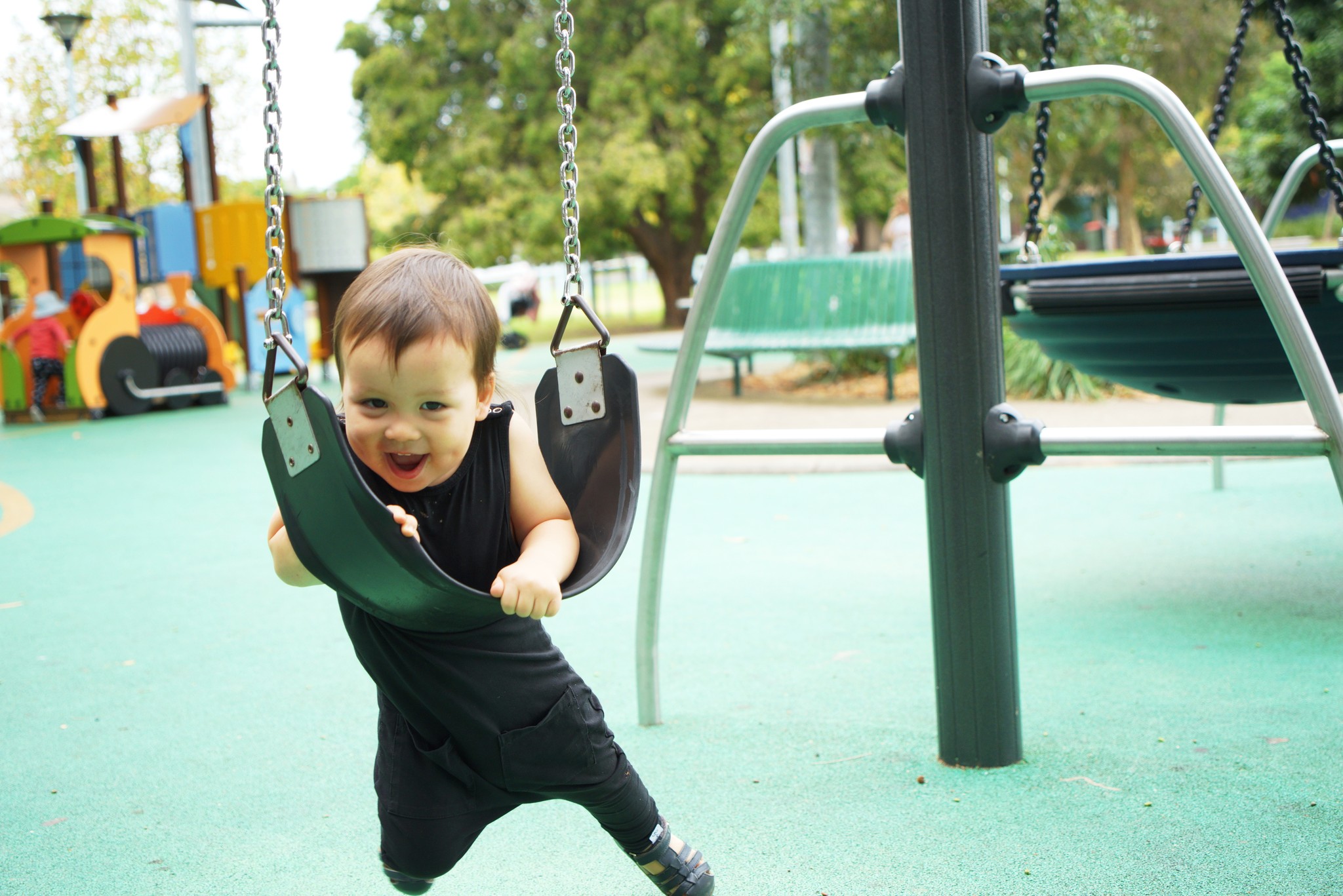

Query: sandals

[614,814,714,896]
[381,862,433,895]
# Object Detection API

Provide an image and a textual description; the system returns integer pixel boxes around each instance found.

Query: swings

[996,0,1343,406]
[260,0,644,636]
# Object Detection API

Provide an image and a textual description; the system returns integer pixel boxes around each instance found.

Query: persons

[269,246,714,896]
[8,291,74,425]
[881,187,911,252]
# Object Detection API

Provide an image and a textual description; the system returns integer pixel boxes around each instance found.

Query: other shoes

[29,407,46,424]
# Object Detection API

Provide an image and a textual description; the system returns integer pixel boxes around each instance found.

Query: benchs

[635,251,916,400]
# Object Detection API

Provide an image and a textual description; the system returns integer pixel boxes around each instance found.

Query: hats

[31,290,68,320]
